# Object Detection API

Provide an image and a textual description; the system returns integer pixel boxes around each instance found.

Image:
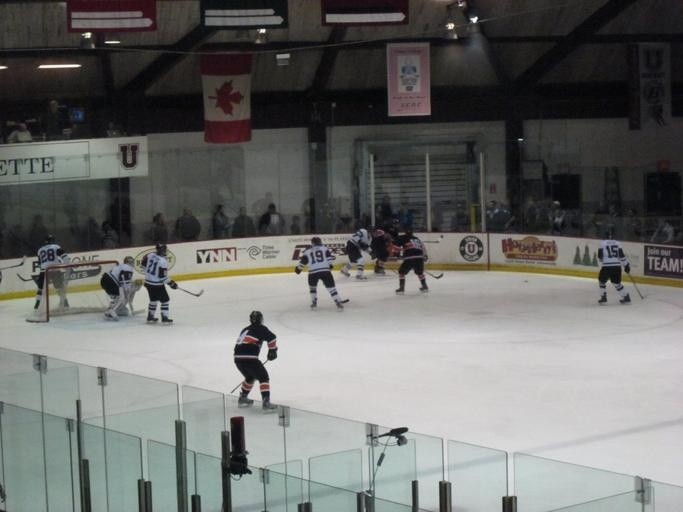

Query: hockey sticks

[177,287,204,297]
[16,272,41,282]
[0,256,27,270]
[121,275,146,315]
[228,359,270,395]
[424,269,444,279]
[627,272,645,301]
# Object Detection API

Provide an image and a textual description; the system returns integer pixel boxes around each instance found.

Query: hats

[552,201,561,206]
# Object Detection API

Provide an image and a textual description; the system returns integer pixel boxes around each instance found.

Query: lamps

[83,33,95,49]
[444,6,455,30]
[256,28,266,43]
[464,7,478,24]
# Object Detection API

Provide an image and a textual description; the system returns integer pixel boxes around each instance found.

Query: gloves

[624,264,630,273]
[295,266,302,274]
[167,280,178,289]
[268,350,277,361]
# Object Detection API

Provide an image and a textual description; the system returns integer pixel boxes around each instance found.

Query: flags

[626,43,674,130]
[387,43,431,116]
[202,55,253,144]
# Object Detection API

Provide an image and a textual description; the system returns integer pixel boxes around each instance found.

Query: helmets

[312,237,322,246]
[406,230,412,237]
[156,242,166,254]
[47,233,55,243]
[607,231,615,240]
[124,256,134,264]
[250,311,263,325]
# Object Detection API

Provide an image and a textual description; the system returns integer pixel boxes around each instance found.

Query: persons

[99,257,136,321]
[301,195,472,234]
[0,212,51,259]
[233,207,257,238]
[172,207,202,243]
[257,203,286,237]
[486,199,614,239]
[340,225,376,281]
[370,221,397,275]
[212,204,236,239]
[295,237,345,311]
[607,205,682,245]
[387,228,428,292]
[69,216,131,251]
[252,191,273,220]
[597,229,631,304]
[144,212,169,245]
[290,215,302,235]
[35,235,69,308]
[233,311,278,410]
[142,243,177,323]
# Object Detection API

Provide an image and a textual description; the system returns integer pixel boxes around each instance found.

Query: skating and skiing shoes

[162,315,173,323]
[335,299,349,308]
[34,306,38,312]
[310,302,317,309]
[620,295,631,304]
[599,297,607,304]
[420,286,428,290]
[147,314,159,322]
[238,392,253,407]
[105,309,120,321]
[263,399,277,410]
[375,267,385,275]
[340,268,350,277]
[396,286,405,294]
[356,272,368,280]
